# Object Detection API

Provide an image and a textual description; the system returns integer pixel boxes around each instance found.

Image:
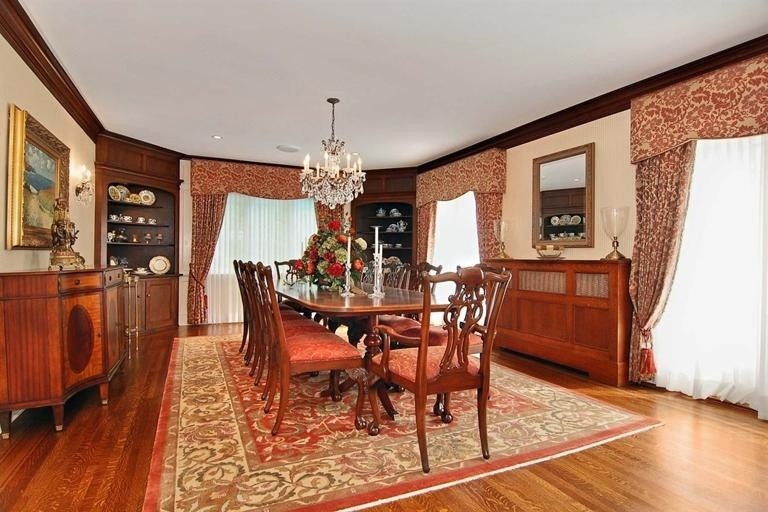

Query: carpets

[141,322,675,512]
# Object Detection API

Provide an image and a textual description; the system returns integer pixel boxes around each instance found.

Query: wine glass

[599,204,630,259]
[492,220,514,261]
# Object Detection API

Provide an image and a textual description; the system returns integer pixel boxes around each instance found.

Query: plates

[108,184,158,207]
[148,255,170,274]
[535,256,567,261]
[550,215,582,225]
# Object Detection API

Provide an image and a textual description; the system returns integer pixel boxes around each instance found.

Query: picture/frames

[4,101,74,252]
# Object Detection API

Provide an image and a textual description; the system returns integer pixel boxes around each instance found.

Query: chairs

[234,253,510,436]
[368,268,511,475]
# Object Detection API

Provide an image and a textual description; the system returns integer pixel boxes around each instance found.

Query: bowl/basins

[536,244,563,257]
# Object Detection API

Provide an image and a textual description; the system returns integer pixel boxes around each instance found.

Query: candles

[378,244,384,274]
[375,226,379,257]
[347,232,352,265]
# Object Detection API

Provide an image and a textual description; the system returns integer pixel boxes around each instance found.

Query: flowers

[295,211,370,279]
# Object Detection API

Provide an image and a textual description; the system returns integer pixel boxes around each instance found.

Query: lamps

[74,163,94,197]
[300,96,368,209]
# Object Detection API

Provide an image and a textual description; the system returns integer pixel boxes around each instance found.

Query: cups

[107,213,164,243]
[550,232,587,239]
[131,268,152,277]
[372,206,408,249]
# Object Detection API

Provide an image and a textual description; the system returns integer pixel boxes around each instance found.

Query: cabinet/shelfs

[95,161,184,274]
[539,209,587,241]
[480,255,632,388]
[123,274,183,337]
[348,190,418,267]
[0,265,128,437]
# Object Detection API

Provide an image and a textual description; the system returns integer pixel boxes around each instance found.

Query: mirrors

[529,140,597,249]
